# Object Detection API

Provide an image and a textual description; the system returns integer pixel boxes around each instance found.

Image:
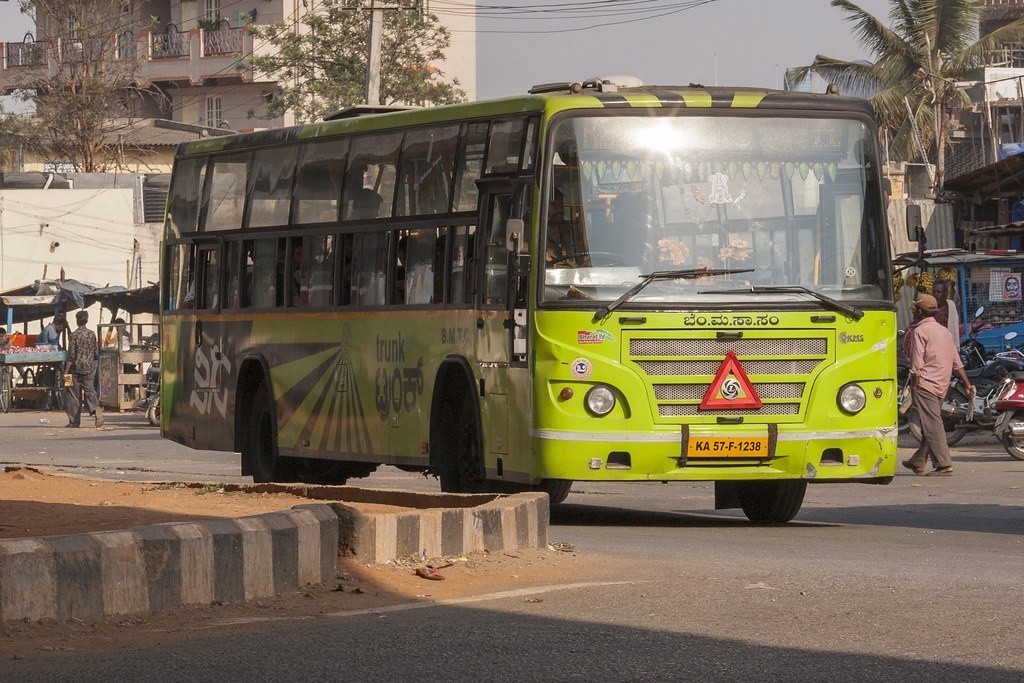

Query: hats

[76,311,88,320]
[915,294,940,311]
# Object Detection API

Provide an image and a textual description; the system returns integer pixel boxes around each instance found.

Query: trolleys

[0,351,69,414]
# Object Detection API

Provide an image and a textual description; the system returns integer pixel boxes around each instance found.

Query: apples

[992,310,1016,321]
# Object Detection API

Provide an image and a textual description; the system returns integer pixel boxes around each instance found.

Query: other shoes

[902,460,926,476]
[926,466,954,477]
[65,423,79,428]
[95,407,104,428]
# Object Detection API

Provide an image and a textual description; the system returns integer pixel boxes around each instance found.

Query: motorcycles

[895,303,1024,463]
[146,359,162,428]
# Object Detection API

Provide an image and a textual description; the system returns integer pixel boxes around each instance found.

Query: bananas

[892,269,956,302]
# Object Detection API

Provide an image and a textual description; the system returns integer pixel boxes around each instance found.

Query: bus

[156,73,930,530]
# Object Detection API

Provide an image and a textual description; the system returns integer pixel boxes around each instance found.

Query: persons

[107,319,129,344]
[36,315,67,343]
[901,292,976,476]
[931,280,960,350]
[523,187,592,265]
[63,311,104,428]
[181,188,474,308]
